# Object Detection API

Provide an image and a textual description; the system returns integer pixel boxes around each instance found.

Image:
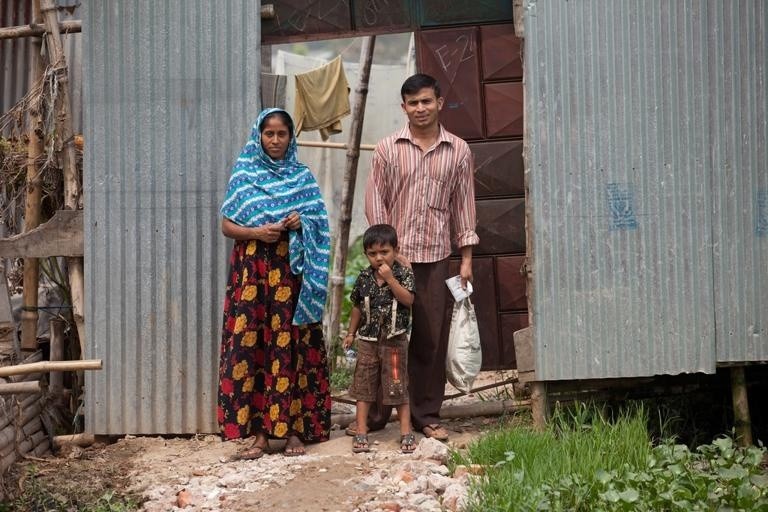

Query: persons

[343,72,482,442]
[215,105,334,461]
[341,222,418,455]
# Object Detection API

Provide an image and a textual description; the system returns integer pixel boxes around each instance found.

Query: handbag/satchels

[446,300,482,395]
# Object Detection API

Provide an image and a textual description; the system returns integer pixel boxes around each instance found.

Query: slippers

[241,445,273,459]
[345,420,373,436]
[419,424,448,440]
[352,434,370,452]
[400,433,417,452]
[283,443,305,456]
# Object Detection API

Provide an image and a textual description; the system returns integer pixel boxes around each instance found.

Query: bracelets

[346,332,357,338]
